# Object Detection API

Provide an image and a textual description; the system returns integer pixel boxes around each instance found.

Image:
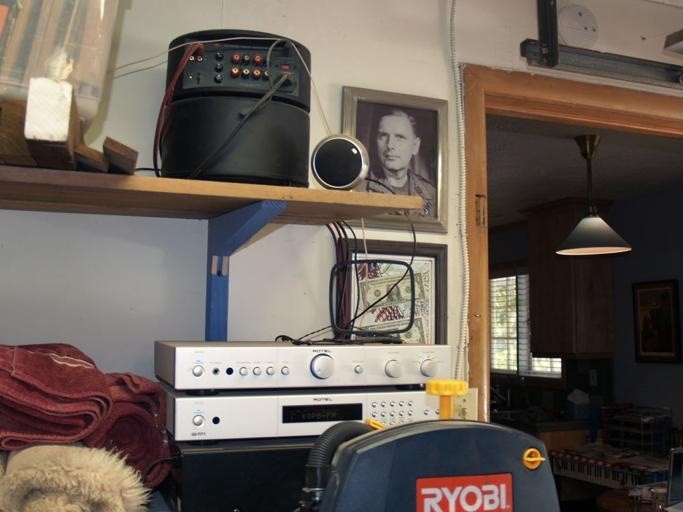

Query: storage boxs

[0,0,124,141]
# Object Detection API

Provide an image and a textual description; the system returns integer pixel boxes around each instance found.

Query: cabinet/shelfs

[534,428,606,504]
[515,199,616,363]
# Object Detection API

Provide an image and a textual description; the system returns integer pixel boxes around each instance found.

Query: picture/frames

[627,277,680,367]
[335,81,451,239]
[333,233,449,346]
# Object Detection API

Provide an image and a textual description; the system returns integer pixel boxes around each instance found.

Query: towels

[0,339,112,453]
[79,371,175,489]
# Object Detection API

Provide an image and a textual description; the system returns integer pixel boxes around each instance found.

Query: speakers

[160,97,310,188]
[311,133,371,189]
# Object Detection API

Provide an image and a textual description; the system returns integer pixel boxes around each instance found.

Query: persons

[385,284,402,303]
[363,111,436,217]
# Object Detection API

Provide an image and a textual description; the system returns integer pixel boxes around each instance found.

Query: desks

[596,487,668,512]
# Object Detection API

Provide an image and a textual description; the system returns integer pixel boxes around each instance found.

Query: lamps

[554,131,634,260]
